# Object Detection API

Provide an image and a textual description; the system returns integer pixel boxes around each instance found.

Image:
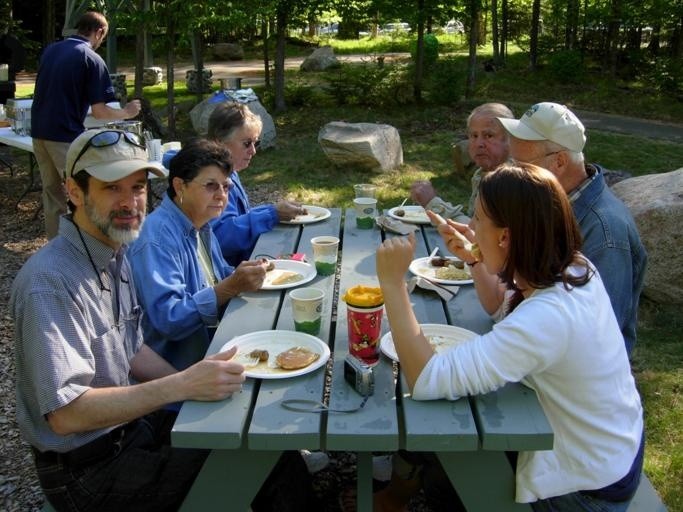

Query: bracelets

[467,259,480,267]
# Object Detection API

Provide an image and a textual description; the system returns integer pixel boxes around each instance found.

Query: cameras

[344,353,374,397]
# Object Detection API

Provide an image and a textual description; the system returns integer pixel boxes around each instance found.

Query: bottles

[341,286,384,367]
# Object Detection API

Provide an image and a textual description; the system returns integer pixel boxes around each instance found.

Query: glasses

[70,130,147,173]
[242,140,261,148]
[200,179,232,192]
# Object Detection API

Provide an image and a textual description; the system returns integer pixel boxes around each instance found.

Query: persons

[335,160,648,512]
[492,99,649,364]
[1,19,27,106]
[28,9,144,243]
[125,137,333,474]
[204,100,309,271]
[7,125,316,512]
[409,102,516,227]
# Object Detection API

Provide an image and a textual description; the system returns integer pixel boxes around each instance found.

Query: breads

[273,272,304,285]
[277,347,321,369]
[435,263,471,280]
[471,242,480,258]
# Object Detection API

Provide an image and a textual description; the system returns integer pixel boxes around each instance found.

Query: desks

[0,125,43,222]
[158,205,558,511]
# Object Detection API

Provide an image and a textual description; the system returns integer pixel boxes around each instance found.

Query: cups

[353,184,377,197]
[354,198,377,228]
[290,287,326,335]
[311,236,340,275]
[146,139,160,161]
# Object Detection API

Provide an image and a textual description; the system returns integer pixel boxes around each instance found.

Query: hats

[495,101,588,153]
[65,128,166,184]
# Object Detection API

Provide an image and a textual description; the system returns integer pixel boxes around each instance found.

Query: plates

[408,257,477,285]
[218,330,330,380]
[280,205,331,225]
[389,206,431,222]
[262,260,318,290]
[380,325,483,364]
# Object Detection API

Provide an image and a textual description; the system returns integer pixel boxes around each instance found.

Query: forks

[435,212,473,253]
[240,357,260,368]
